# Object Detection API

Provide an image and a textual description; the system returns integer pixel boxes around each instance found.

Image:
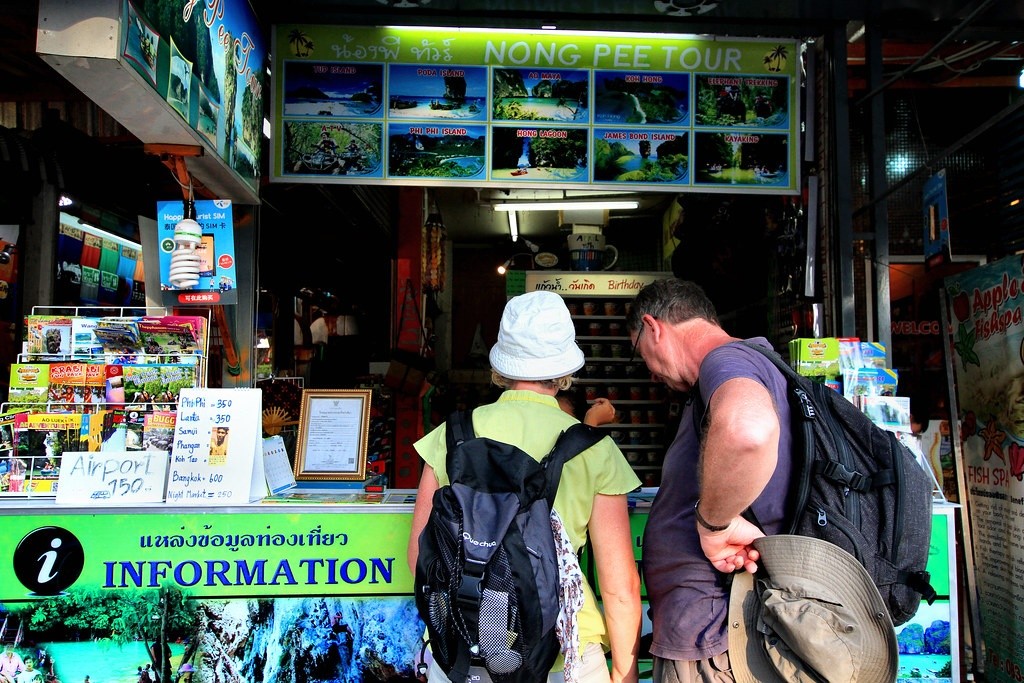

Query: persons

[623,270,840,683]
[555,380,616,433]
[407,289,643,683]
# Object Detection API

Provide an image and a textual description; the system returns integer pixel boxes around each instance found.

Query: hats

[728,535,899,683]
[179,663,195,672]
[489,290,585,380]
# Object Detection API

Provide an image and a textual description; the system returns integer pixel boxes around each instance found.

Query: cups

[567,233,617,272]
[566,302,660,488]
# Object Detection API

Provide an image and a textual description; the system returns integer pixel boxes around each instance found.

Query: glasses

[630,316,657,367]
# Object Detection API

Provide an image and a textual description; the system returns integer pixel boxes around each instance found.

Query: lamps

[491,198,641,211]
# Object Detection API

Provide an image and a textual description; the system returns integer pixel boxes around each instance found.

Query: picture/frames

[293,389,372,480]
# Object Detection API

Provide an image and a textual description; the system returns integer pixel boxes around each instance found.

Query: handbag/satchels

[385,348,432,395]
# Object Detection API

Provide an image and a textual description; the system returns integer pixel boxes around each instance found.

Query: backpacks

[413,405,612,683]
[694,339,938,626]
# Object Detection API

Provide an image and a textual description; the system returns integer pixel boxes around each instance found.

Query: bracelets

[694,497,732,532]
